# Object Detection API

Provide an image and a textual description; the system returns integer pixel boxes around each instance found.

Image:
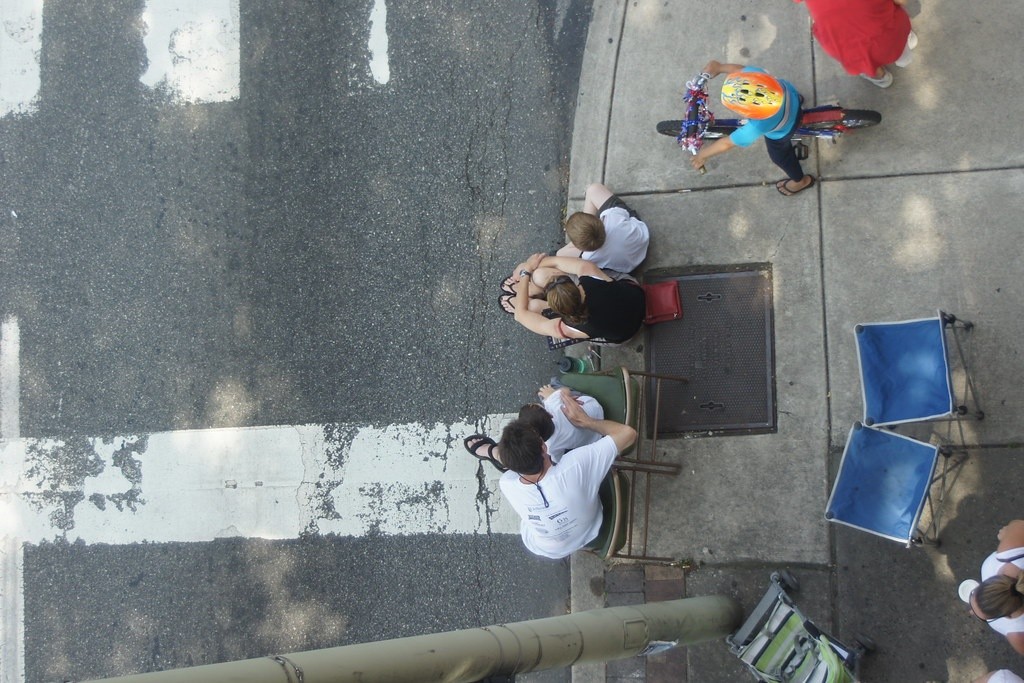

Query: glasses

[544,275,574,296]
[968,589,997,624]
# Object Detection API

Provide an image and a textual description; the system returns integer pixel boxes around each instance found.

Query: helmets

[719,67,785,119]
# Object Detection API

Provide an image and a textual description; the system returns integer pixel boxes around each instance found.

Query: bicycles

[654,72,882,175]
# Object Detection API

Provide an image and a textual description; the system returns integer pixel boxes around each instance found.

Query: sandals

[464,435,508,474]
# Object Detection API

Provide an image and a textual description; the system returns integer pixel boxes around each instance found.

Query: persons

[463,392,638,560]
[794,0,920,89]
[497,251,647,348]
[688,59,816,196]
[968,668,1024,683]
[958,519,1024,657]
[554,182,651,274]
[518,377,605,466]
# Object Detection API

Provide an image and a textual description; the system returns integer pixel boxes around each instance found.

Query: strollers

[723,569,877,683]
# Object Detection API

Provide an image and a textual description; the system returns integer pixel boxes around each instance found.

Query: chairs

[554,366,691,469]
[581,466,681,562]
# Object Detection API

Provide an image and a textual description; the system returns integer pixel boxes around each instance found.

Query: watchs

[519,269,531,278]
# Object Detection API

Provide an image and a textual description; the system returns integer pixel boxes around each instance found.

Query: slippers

[499,276,518,294]
[497,294,518,315]
[775,174,814,194]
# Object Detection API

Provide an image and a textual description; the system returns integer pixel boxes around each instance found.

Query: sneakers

[856,67,893,89]
[894,46,914,67]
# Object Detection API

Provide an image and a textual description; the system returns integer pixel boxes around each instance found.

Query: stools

[853,308,986,431]
[824,422,953,550]
